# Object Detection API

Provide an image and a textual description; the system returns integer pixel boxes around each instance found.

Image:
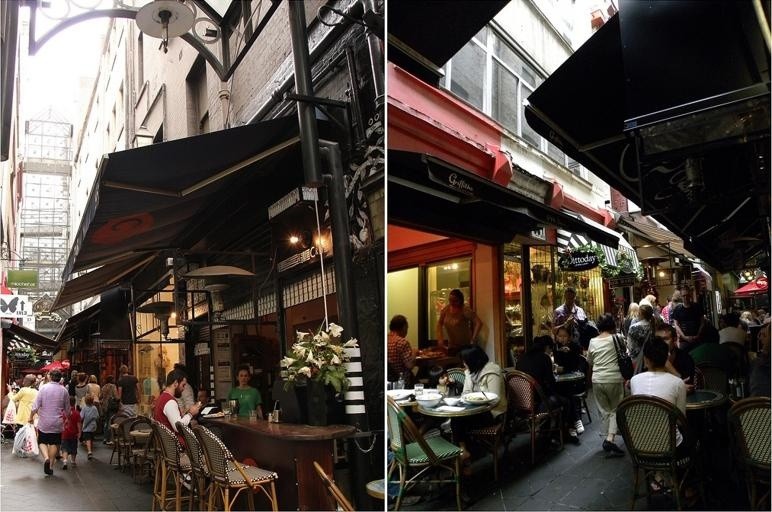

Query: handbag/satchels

[630,332,649,376]
[609,334,635,379]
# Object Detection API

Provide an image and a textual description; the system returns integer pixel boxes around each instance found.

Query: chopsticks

[481,390,490,401]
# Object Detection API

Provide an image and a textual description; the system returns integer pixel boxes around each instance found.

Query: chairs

[131,419,157,484]
[118,417,141,476]
[573,354,593,425]
[702,368,730,396]
[727,396,771,511]
[194,425,278,510]
[443,374,510,481]
[444,367,465,388]
[145,417,220,511]
[616,394,698,511]
[386,398,464,511]
[504,370,565,464]
[109,413,130,469]
[315,460,355,510]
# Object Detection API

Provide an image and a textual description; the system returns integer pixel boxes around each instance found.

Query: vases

[307,379,327,427]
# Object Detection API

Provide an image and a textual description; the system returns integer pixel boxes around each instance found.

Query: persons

[118,364,142,418]
[436,288,483,368]
[630,336,720,498]
[450,343,508,475]
[226,365,263,419]
[645,295,656,305]
[6,374,39,436]
[552,287,587,340]
[75,372,88,445]
[748,364,771,397]
[626,305,664,373]
[697,320,729,375]
[661,296,672,321]
[655,323,697,395]
[586,314,640,447]
[68,369,79,396]
[751,310,764,325]
[174,363,194,415]
[668,292,682,323]
[428,364,464,400]
[387,314,416,373]
[12,376,21,390]
[28,368,72,476]
[621,302,639,334]
[80,395,100,460]
[154,369,203,491]
[672,287,705,353]
[197,388,210,413]
[718,315,727,329]
[719,312,747,345]
[35,374,42,391]
[60,396,83,470]
[86,375,100,402]
[99,375,119,445]
[655,303,661,313]
[3,387,18,419]
[516,335,580,446]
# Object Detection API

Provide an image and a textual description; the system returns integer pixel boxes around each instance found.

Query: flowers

[279,322,360,394]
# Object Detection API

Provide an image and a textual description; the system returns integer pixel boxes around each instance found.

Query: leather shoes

[602,440,625,456]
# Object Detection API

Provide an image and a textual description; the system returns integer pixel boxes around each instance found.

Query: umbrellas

[733,275,769,308]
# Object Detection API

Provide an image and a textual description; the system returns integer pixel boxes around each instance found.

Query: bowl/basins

[443,398,460,406]
[414,393,442,408]
[387,389,414,400]
[423,389,440,394]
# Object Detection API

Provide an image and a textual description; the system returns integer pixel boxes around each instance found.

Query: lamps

[136,1,194,54]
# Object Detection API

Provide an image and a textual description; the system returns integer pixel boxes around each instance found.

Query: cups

[414,384,424,395]
[248,409,257,423]
[223,407,231,421]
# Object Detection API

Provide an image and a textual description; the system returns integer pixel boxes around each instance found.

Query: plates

[463,392,498,405]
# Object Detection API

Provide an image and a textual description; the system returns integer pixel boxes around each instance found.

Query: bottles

[396,372,406,389]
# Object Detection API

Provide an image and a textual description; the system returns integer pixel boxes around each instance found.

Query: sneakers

[88,453,93,460]
[569,428,578,438]
[63,462,67,470]
[44,460,50,473]
[574,420,585,434]
